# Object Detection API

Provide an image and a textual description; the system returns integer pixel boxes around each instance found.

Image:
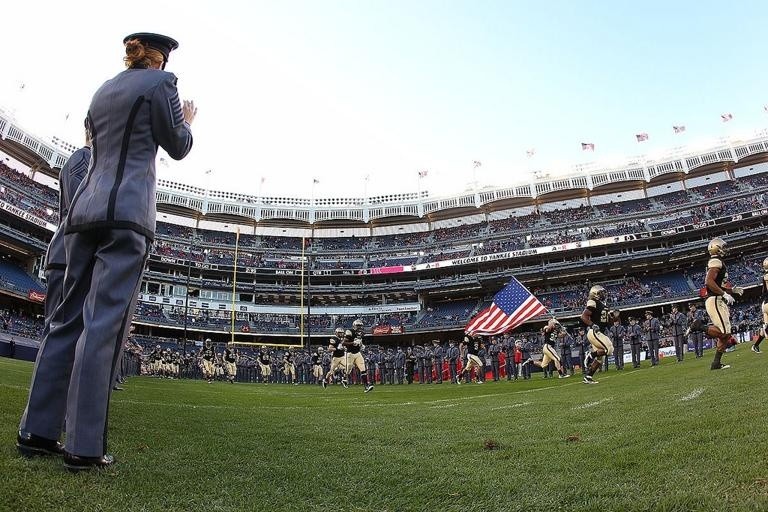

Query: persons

[15,31,198,473]
[0,158,768,395]
[41,131,91,431]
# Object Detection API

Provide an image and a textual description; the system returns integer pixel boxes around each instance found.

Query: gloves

[338,344,348,352]
[722,291,736,306]
[590,323,600,333]
[353,338,365,352]
[732,284,744,296]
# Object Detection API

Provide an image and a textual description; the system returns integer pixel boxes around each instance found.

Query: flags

[636,133,649,142]
[673,126,685,134]
[721,113,732,122]
[581,142,595,153]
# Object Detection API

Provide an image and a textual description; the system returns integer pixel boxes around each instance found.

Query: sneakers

[584,350,594,370]
[583,375,599,385]
[152,374,179,381]
[380,379,414,384]
[684,319,700,338]
[493,376,532,382]
[251,379,299,386]
[751,344,763,355]
[521,358,534,367]
[303,379,374,393]
[207,374,236,384]
[615,363,659,371]
[419,373,487,384]
[710,363,732,370]
[542,371,576,379]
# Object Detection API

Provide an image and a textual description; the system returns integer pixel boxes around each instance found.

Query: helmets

[335,328,344,334]
[588,285,608,303]
[262,345,267,350]
[228,342,234,347]
[318,346,323,354]
[205,338,212,345]
[353,320,364,327]
[707,238,729,257]
[763,257,768,273]
[156,345,180,357]
[289,345,295,350]
[190,350,196,356]
[549,318,559,328]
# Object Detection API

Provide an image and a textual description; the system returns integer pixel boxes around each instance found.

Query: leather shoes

[16,427,65,458]
[63,450,115,473]
[113,387,123,391]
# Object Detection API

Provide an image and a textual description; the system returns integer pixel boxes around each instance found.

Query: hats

[492,332,527,340]
[369,339,458,353]
[628,302,696,322]
[123,32,179,62]
